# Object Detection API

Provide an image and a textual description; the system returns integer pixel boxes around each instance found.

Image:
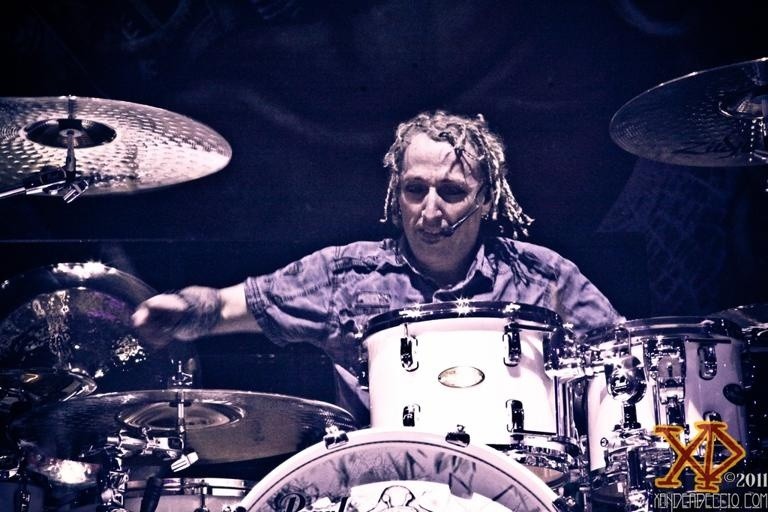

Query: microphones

[442,197,485,237]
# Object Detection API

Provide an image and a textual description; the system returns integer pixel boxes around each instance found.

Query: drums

[235,428,564,510]
[577,318,752,488]
[362,301,575,486]
[63,476,260,512]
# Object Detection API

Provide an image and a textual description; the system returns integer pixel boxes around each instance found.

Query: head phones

[481,176,498,204]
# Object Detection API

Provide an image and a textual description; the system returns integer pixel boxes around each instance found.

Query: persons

[120,108,627,429]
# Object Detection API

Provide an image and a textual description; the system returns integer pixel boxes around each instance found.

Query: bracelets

[171,283,221,344]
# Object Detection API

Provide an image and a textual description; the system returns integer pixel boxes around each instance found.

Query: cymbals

[16,389,355,461]
[0,97,232,195]
[2,263,174,488]
[611,59,766,166]
